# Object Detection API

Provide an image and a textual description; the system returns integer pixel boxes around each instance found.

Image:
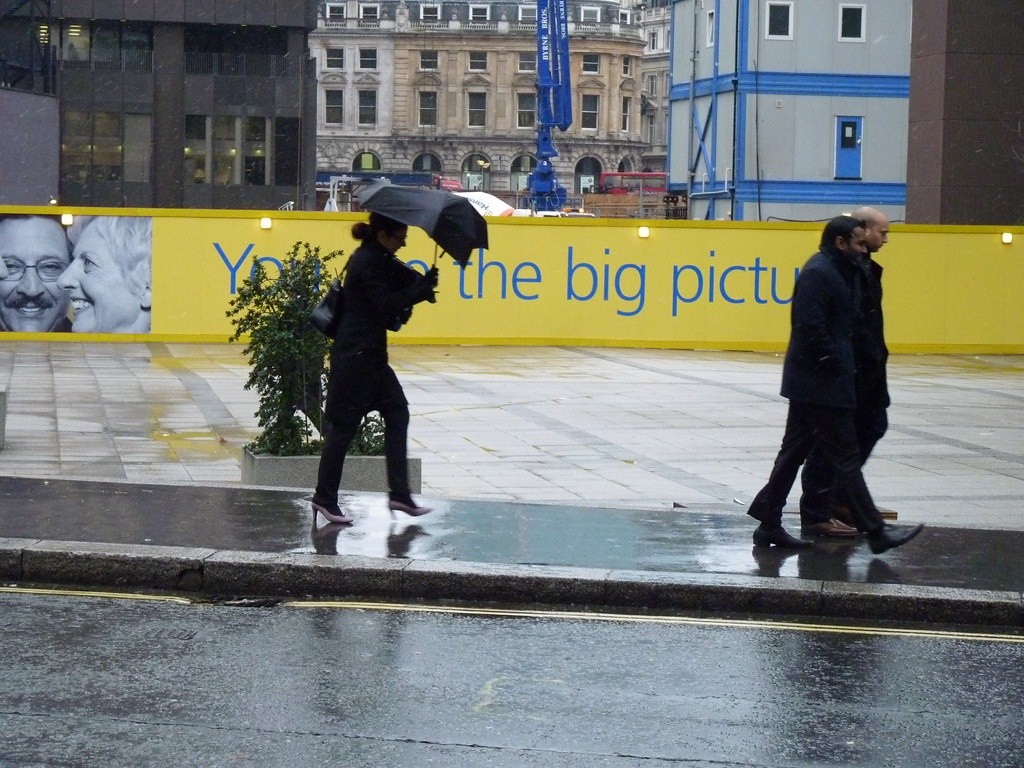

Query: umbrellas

[356,183,489,270]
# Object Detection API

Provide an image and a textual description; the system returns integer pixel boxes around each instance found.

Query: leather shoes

[803,505,860,536]
[868,523,924,554]
[753,525,814,548]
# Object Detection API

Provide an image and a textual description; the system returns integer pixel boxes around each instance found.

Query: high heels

[311,502,353,525]
[388,501,432,520]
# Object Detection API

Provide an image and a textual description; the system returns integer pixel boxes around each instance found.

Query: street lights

[477,160,490,190]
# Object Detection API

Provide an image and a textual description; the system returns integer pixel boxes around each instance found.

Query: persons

[310,212,439,530]
[747,207,924,555]
[0,213,151,333]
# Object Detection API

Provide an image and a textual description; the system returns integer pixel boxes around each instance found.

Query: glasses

[392,234,407,242]
[2,256,68,282]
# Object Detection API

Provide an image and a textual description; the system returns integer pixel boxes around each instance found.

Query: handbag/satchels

[308,247,361,338]
